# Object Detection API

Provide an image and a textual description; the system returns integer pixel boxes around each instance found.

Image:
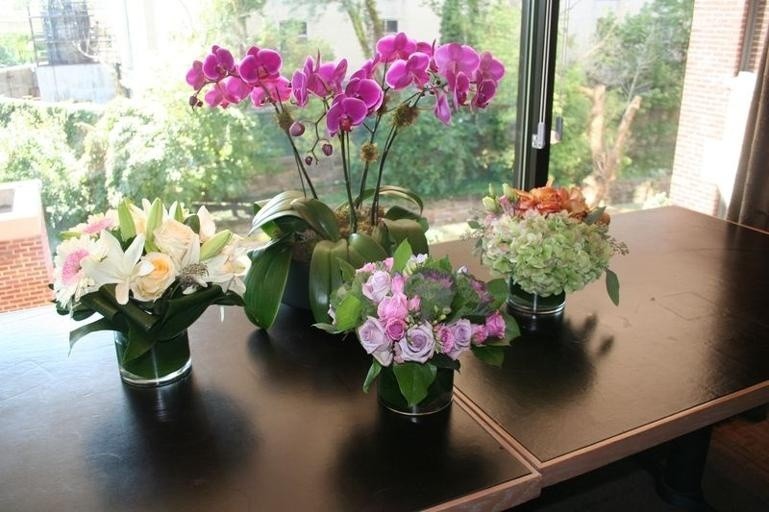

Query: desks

[0,277,545,512]
[417,201,768,507]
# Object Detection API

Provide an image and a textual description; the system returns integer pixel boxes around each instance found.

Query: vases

[375,366,455,418]
[112,328,194,390]
[504,276,568,333]
[279,240,312,313]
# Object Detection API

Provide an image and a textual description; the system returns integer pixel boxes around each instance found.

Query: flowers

[29,196,273,368]
[305,235,522,409]
[460,181,631,306]
[182,31,508,335]
